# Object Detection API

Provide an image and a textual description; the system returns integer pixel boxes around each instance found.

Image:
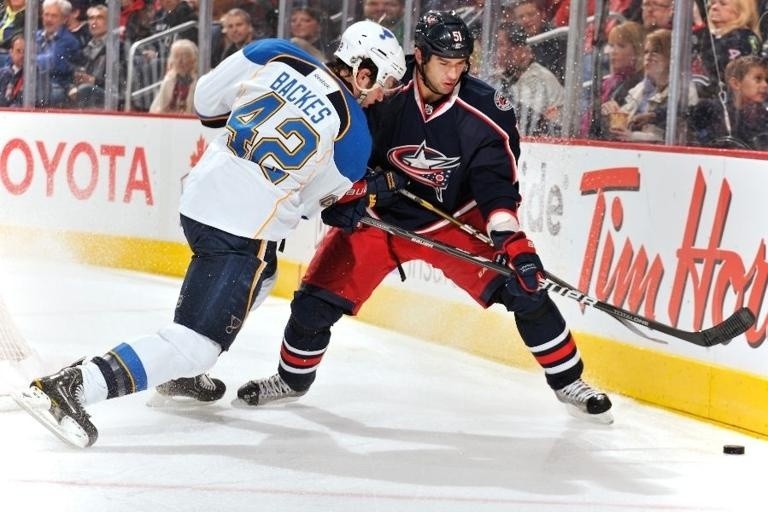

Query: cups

[608,112,629,133]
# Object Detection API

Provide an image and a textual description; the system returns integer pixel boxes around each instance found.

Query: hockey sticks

[362,218,755,348]
[394,189,674,345]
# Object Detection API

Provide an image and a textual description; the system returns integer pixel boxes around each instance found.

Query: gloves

[487,217,550,298]
[320,170,406,231]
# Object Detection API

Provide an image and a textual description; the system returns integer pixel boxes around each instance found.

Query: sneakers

[554,377,612,415]
[237,375,309,405]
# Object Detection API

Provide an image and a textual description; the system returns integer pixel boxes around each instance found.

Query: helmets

[414,10,474,68]
[335,20,407,91]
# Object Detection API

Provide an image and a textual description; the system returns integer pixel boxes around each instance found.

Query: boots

[156,375,226,402]
[29,363,99,450]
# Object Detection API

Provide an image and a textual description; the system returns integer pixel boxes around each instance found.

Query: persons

[0,1,198,108]
[31,21,407,449]
[291,10,325,57]
[456,0,768,152]
[148,39,199,114]
[239,10,612,414]
[363,1,403,46]
[210,0,279,69]
